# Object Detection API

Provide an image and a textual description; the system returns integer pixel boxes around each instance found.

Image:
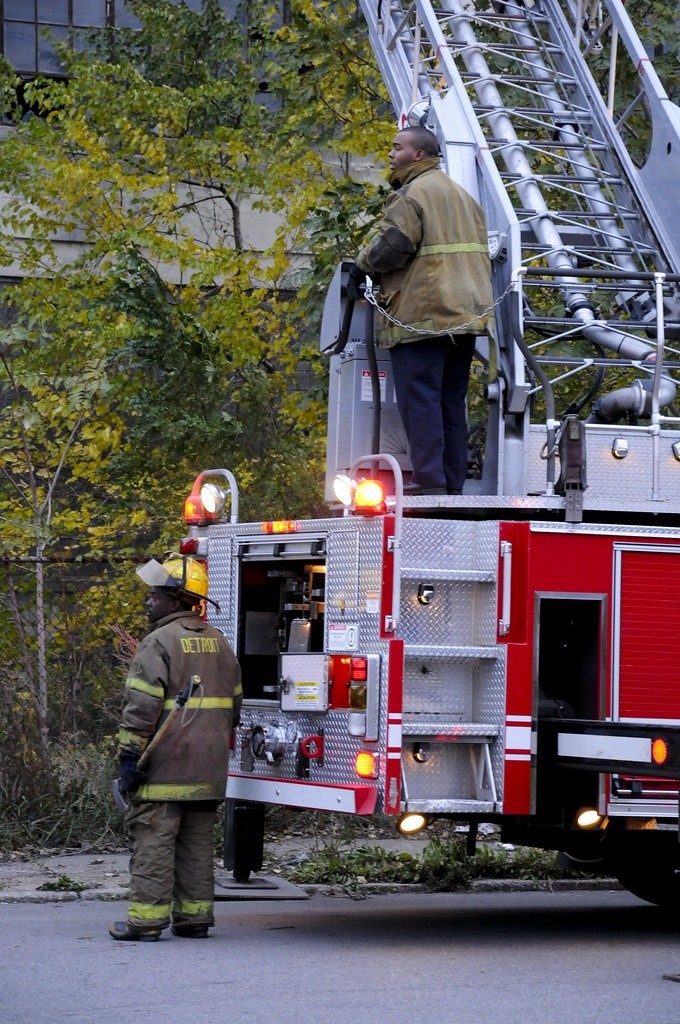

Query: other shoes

[401,478,462,495]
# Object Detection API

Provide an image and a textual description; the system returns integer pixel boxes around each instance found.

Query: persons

[349,125,494,496]
[106,553,243,940]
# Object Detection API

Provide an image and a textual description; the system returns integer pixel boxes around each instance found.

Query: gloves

[119,751,149,793]
[348,265,374,299]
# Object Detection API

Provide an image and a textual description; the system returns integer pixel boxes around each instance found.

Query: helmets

[137,550,208,601]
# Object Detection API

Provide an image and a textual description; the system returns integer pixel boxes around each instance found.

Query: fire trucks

[172,0,680,918]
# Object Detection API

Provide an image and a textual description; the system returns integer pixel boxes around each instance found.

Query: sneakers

[170,922,207,938]
[108,919,161,942]
